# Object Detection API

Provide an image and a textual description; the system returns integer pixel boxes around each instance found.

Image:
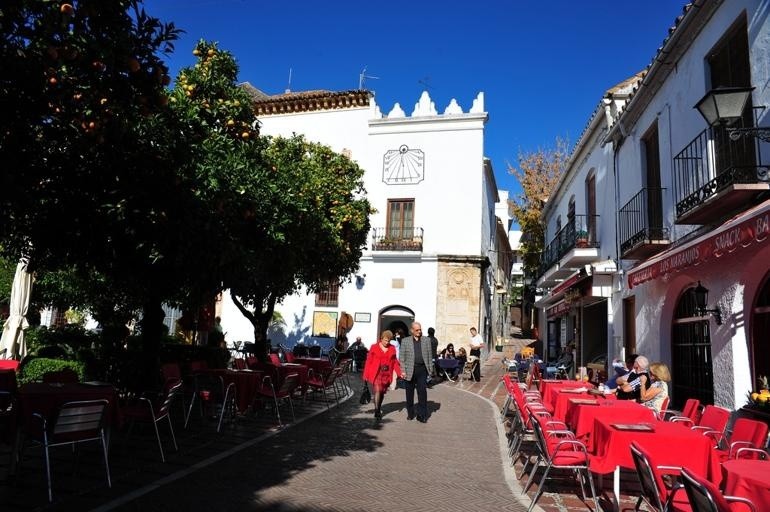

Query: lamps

[694,279,725,328]
[690,83,769,146]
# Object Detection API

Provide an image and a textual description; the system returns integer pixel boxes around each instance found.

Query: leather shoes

[417,416,426,423]
[407,416,413,420]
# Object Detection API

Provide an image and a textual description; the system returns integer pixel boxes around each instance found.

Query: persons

[399,322,433,423]
[215,316,222,332]
[453,347,466,381]
[427,328,438,359]
[439,343,455,359]
[468,327,484,382]
[362,329,399,421]
[546,346,573,380]
[605,354,672,419]
[348,337,363,350]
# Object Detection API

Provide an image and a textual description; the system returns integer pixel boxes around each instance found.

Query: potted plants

[574,230,590,248]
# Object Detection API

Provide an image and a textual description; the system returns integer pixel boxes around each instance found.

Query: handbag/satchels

[360,386,370,404]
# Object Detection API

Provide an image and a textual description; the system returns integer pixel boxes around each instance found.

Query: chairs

[432,345,481,385]
[1,344,356,512]
[501,348,768,512]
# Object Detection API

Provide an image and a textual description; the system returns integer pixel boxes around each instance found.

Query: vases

[496,345,503,352]
